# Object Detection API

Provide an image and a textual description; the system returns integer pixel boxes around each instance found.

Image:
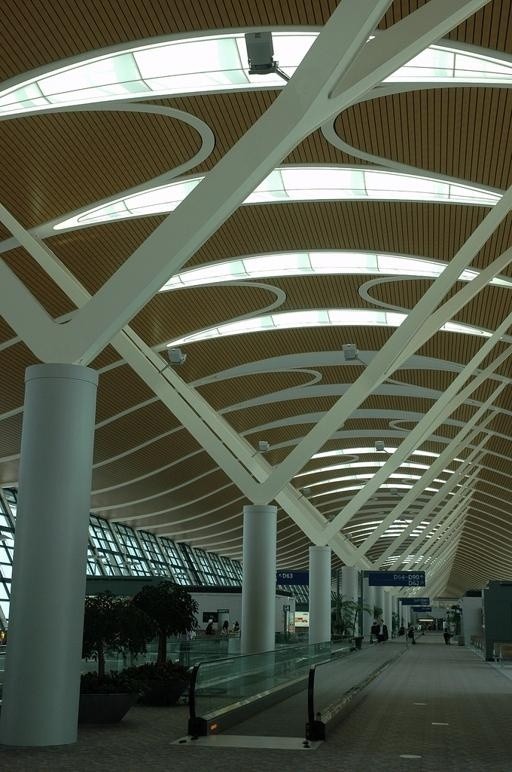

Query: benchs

[470,634,512,669]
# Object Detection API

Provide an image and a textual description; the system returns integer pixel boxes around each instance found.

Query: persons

[205,619,239,636]
[443,620,448,632]
[371,618,415,642]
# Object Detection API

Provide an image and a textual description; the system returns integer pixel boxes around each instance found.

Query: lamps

[244,31,290,83]
[375,441,391,457]
[341,342,369,371]
[158,343,188,374]
[249,440,271,459]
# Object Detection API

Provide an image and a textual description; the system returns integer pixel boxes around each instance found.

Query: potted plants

[124,580,198,707]
[79,589,142,726]
